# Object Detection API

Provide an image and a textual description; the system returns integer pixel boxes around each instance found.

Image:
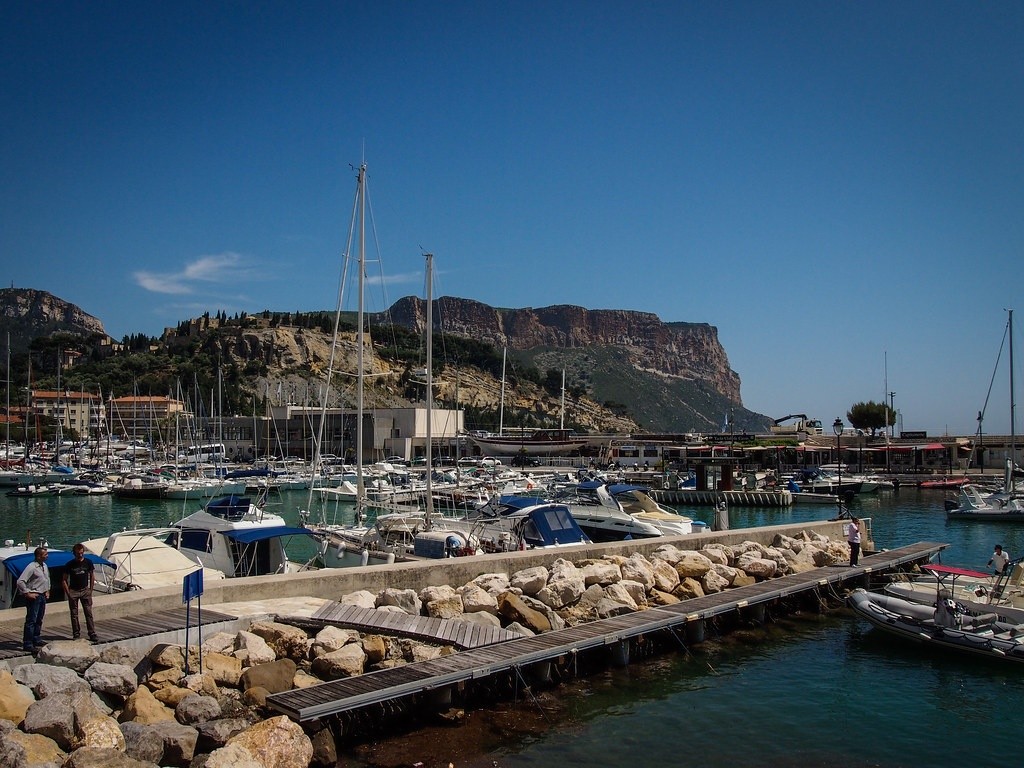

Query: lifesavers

[527,483,532,489]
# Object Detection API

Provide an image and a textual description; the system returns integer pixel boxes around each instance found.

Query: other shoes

[23,647,40,653]
[34,641,48,646]
[856,563,861,566]
[88,631,99,641]
[73,636,80,641]
[851,564,857,568]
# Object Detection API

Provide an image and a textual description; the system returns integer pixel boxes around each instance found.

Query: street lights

[832,417,844,521]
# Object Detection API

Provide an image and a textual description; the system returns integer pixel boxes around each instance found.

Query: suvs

[247,451,345,466]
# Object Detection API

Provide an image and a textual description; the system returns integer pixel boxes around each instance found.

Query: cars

[379,455,503,467]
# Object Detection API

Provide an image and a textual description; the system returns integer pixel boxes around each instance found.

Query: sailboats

[943,309,1024,519]
[461,347,589,455]
[1,333,372,499]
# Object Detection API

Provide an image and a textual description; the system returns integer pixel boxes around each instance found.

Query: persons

[16,547,51,652]
[847,516,862,567]
[61,544,100,642]
[986,544,1009,576]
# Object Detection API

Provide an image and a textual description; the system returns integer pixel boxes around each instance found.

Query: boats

[915,562,1024,586]
[0,131,717,611]
[844,587,1024,664]
[660,445,970,496]
[884,581,1024,624]
